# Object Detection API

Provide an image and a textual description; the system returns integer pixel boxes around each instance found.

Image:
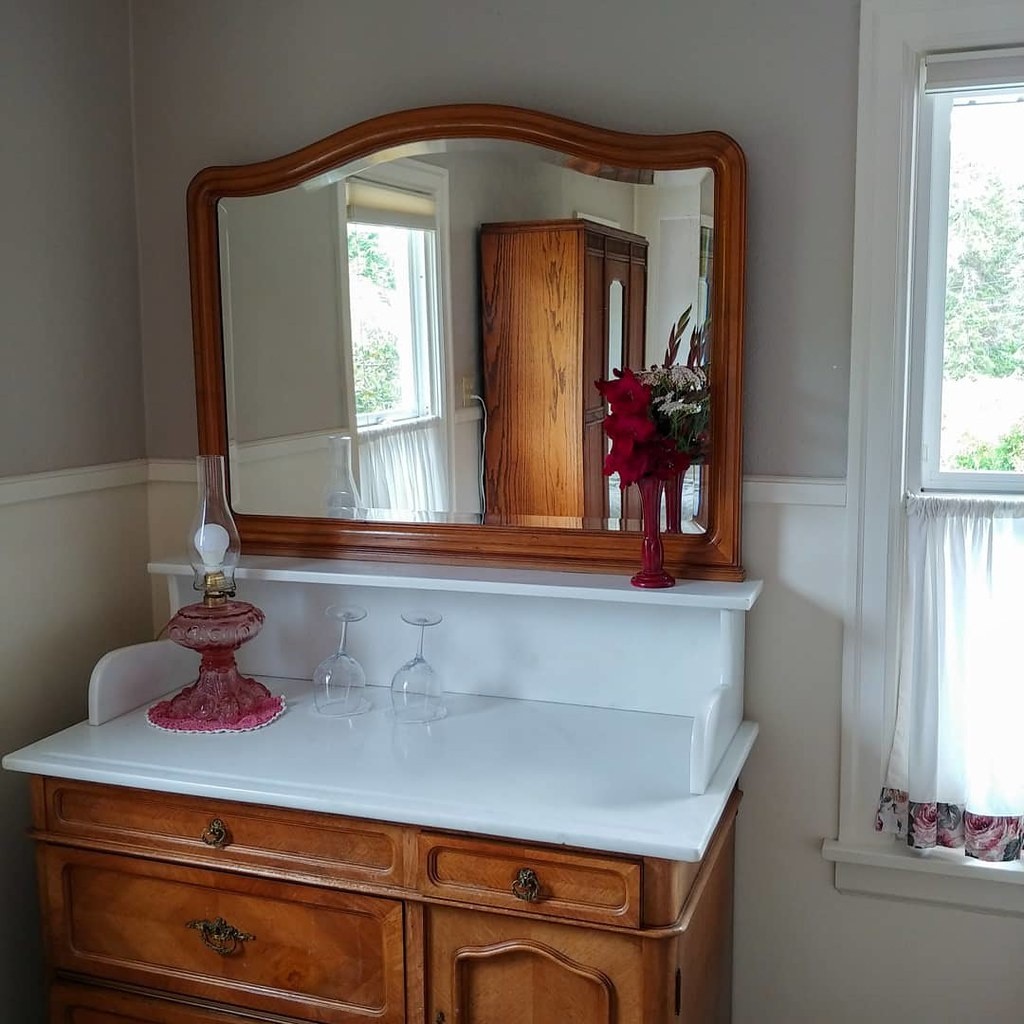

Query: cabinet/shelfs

[478,220,648,527]
[25,774,742,1024]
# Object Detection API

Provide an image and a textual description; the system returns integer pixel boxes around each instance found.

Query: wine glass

[391,609,446,723]
[313,605,368,715]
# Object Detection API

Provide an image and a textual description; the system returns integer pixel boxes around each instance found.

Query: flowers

[593,303,709,489]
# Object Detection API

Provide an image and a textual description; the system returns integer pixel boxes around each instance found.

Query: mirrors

[184,103,749,581]
[607,279,628,519]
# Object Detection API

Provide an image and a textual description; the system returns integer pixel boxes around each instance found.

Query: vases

[631,477,675,588]
[664,470,687,534]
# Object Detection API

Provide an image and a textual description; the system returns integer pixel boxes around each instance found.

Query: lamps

[144,455,288,734]
